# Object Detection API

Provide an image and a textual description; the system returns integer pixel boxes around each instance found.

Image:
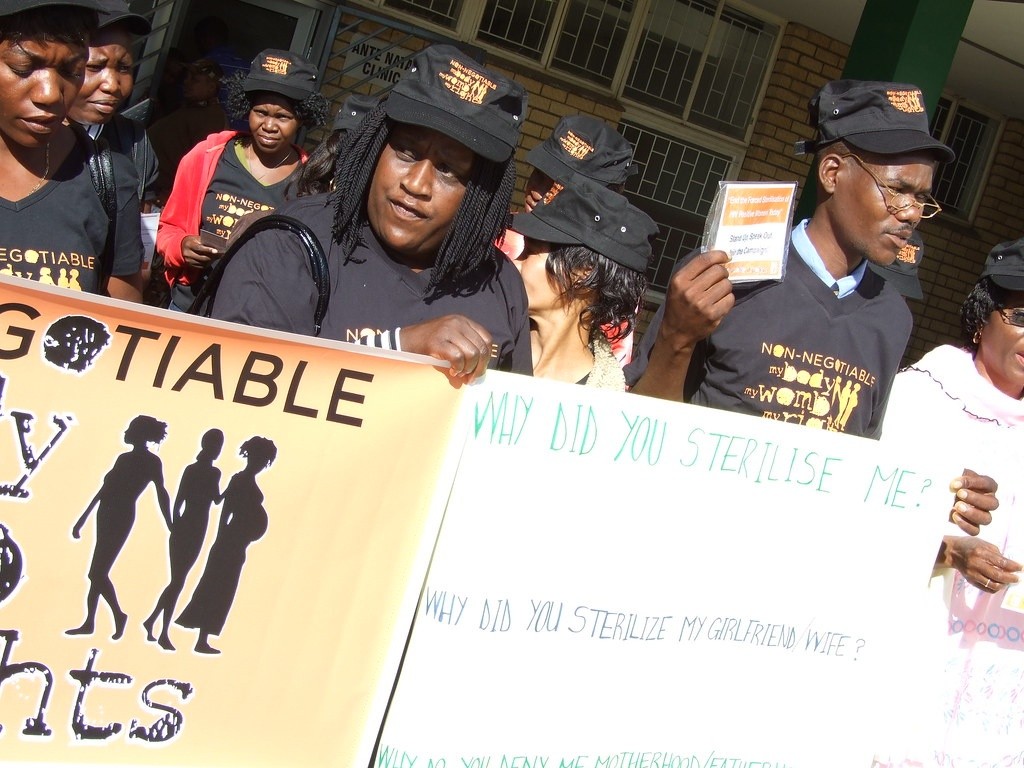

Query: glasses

[997,295,1024,326]
[839,153,942,218]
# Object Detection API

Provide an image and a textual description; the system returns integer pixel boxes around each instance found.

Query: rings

[985,579,990,588]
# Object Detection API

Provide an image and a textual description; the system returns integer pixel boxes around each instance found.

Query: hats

[792,78,955,164]
[980,239,1024,291]
[386,45,528,163]
[96,1,151,37]
[243,49,319,101]
[507,172,660,274]
[331,93,374,131]
[868,230,924,304]
[529,115,639,191]
[1,0,109,20]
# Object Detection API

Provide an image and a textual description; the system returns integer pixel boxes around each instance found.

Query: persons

[0,0,1024,594]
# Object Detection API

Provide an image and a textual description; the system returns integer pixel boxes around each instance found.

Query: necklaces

[28,143,50,196]
[249,146,292,180]
